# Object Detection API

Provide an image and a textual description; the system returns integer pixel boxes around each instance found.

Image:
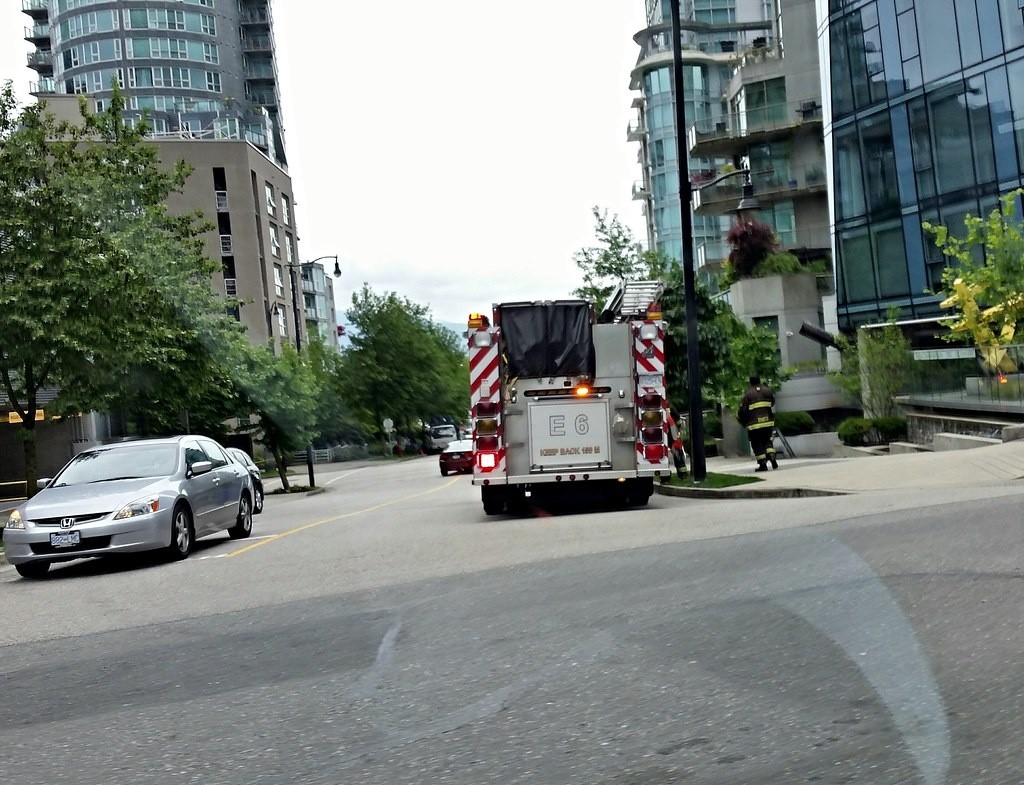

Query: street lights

[283,254,340,483]
[677,168,763,480]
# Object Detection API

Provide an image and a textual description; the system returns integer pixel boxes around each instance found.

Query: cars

[438,439,474,476]
[223,447,265,513]
[2,435,255,578]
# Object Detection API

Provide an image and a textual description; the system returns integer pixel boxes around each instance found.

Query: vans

[430,424,457,453]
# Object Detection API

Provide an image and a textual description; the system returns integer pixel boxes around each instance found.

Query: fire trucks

[463,299,672,517]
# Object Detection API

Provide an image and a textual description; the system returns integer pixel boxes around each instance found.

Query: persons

[738,373,778,471]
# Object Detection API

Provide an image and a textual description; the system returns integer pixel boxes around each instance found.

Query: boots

[755,463,768,471]
[769,458,779,469]
